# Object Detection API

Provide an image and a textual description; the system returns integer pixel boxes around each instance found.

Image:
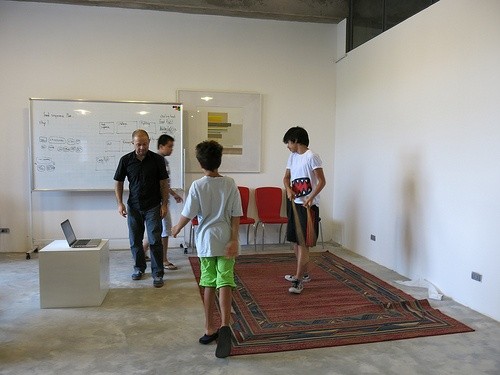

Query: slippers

[216,326,231,357]
[199,328,219,344]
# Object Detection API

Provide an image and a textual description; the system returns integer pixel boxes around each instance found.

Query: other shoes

[164,263,177,270]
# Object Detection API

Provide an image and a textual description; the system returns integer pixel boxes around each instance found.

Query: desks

[38,238,109,308]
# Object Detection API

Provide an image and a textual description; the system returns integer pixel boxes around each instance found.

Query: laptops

[61,219,101,248]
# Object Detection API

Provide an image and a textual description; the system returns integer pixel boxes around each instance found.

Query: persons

[283,126,326,293]
[144,134,183,270]
[171,140,244,359]
[113,129,170,288]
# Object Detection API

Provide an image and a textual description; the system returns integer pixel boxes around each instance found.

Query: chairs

[255,187,295,249]
[238,187,256,248]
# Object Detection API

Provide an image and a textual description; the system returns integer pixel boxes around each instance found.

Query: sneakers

[289,278,305,294]
[285,272,311,282]
[152,275,164,287]
[132,268,146,279]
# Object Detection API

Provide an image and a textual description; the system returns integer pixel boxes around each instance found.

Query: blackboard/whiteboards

[29,97,184,192]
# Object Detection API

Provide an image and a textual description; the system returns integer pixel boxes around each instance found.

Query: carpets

[188,250,476,357]
